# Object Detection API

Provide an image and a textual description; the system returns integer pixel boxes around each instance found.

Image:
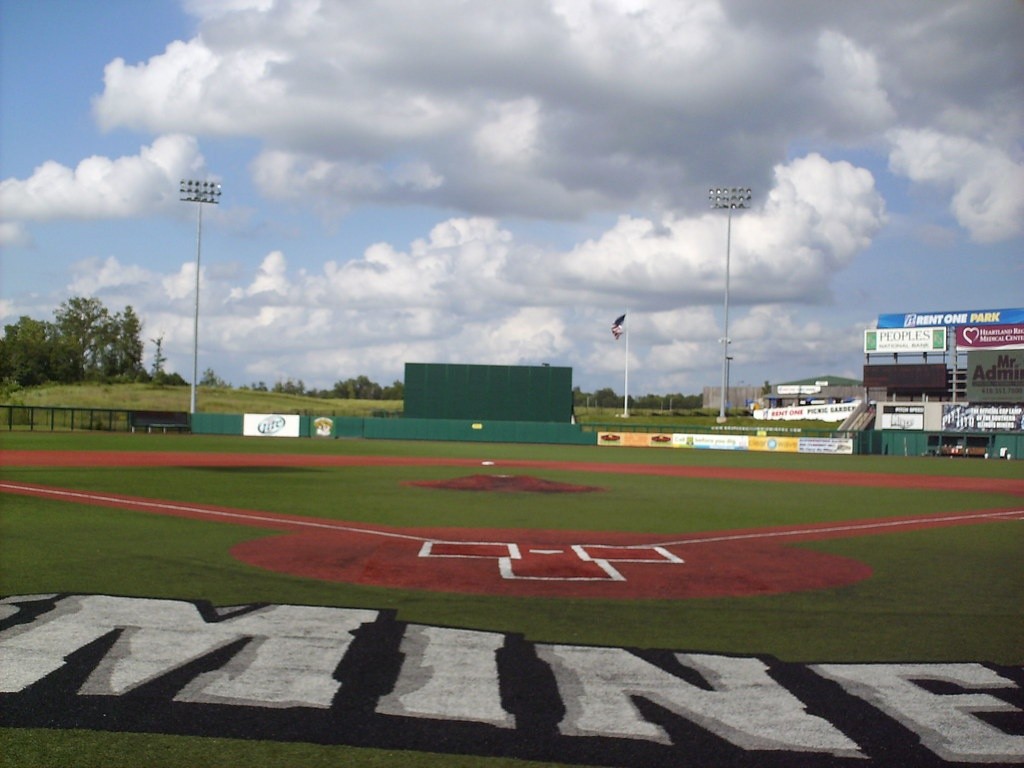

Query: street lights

[180,177,223,410]
[707,185,754,421]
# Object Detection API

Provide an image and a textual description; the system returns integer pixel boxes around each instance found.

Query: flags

[611,314,627,340]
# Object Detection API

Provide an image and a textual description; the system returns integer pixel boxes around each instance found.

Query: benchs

[935,445,986,459]
[130,410,190,435]
